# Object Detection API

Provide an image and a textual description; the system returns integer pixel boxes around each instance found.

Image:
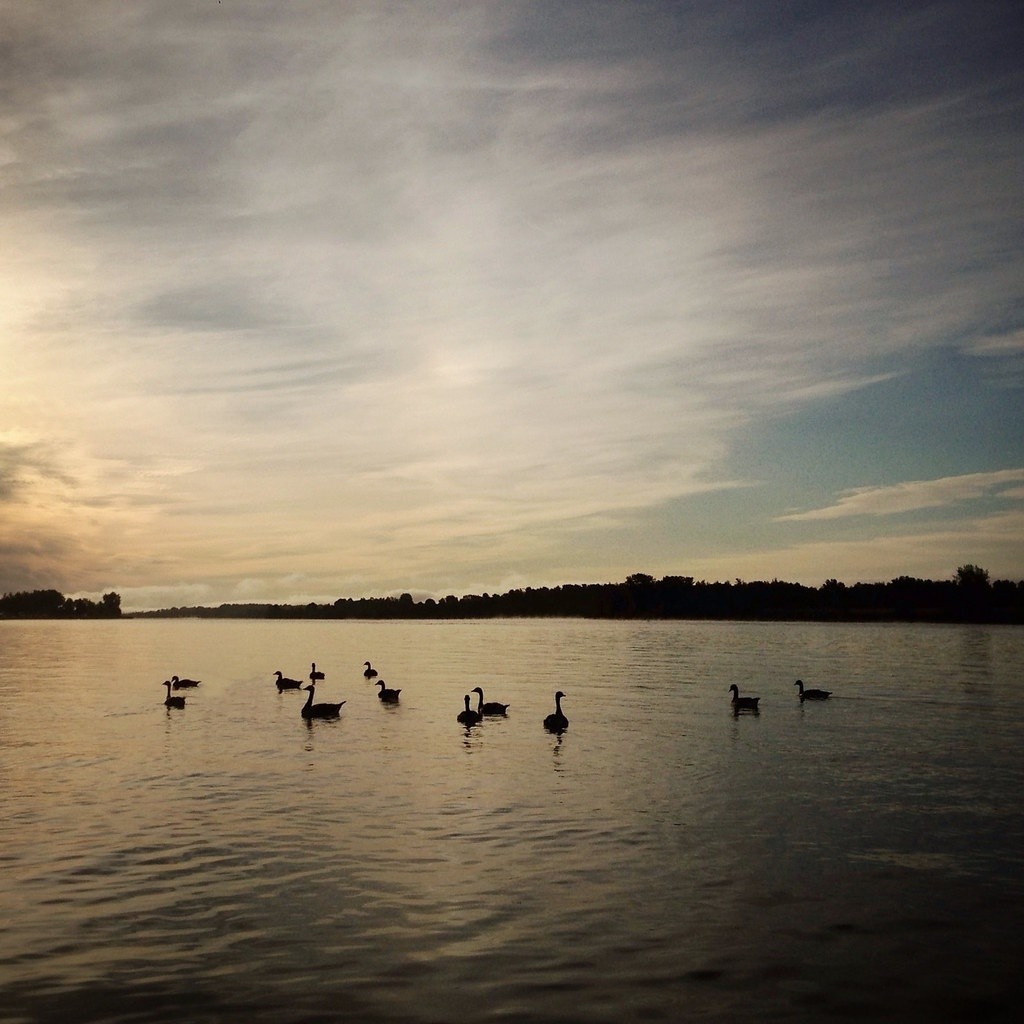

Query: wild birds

[727,684,761,709]
[471,687,510,716]
[273,671,303,691]
[163,680,185,709]
[457,695,481,729]
[309,663,325,680]
[375,680,402,703]
[543,691,569,730]
[171,676,201,691]
[300,685,346,720]
[363,661,378,680]
[793,679,834,700]
